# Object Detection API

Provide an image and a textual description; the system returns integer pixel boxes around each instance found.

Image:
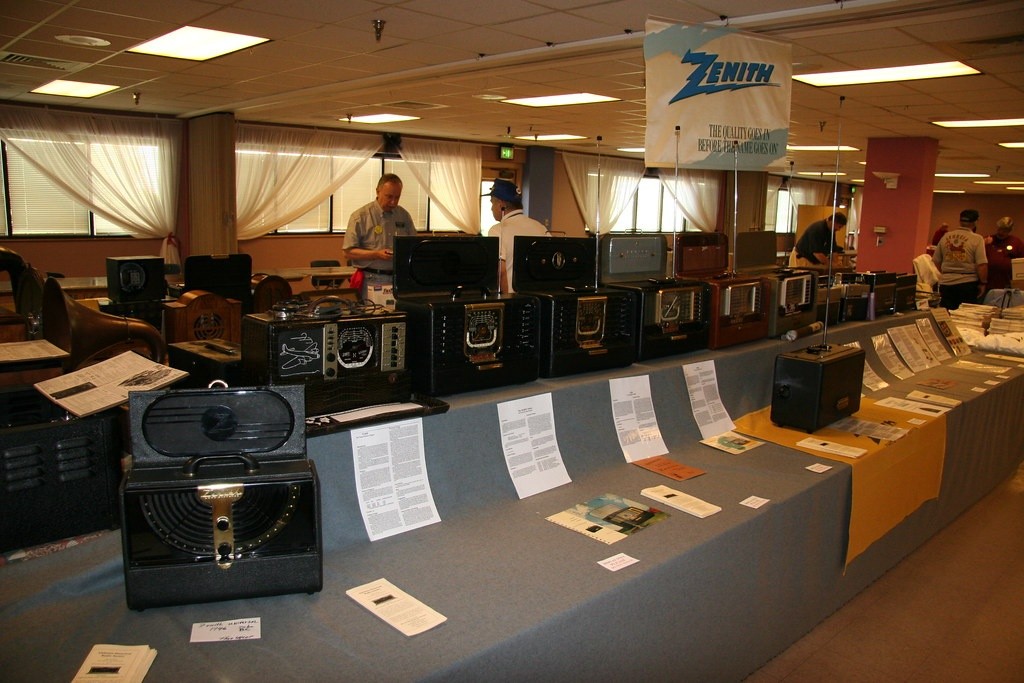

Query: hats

[481,178,520,203]
[960,209,978,222]
[996,216,1013,228]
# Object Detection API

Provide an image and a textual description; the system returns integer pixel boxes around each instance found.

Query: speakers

[769,95,865,435]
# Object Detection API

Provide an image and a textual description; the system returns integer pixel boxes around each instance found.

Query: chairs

[310,260,344,291]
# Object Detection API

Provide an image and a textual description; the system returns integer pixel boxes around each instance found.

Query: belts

[360,268,394,276]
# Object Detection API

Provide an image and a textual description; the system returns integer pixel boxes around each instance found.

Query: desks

[0,276,112,297]
[776,250,858,258]
[0,306,1024,683]
[251,265,358,281]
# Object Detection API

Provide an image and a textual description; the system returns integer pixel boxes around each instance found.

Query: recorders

[240,126,916,419]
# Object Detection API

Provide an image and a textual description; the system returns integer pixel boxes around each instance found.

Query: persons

[932,209,988,316]
[342,173,417,305]
[927,223,949,256]
[488,178,553,294]
[789,212,847,273]
[984,217,1024,301]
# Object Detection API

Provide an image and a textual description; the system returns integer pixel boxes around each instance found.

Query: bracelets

[981,282,987,286]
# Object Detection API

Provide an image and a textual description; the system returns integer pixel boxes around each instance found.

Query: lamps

[872,171,900,183]
